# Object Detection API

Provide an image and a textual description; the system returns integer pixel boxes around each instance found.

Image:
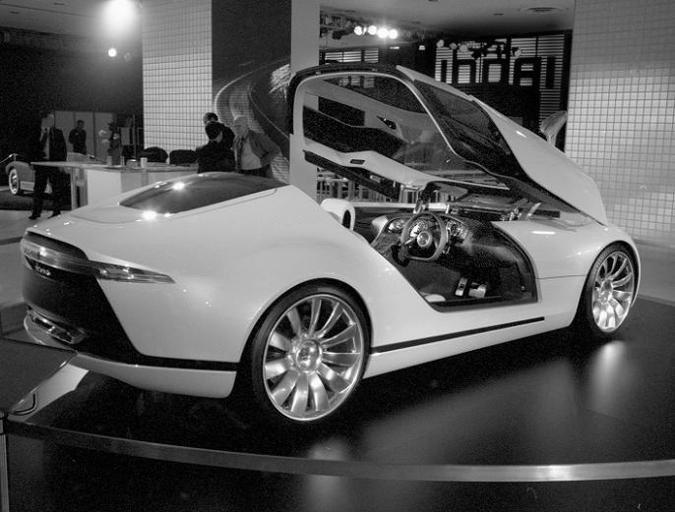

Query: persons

[68,119,88,155]
[197,112,280,179]
[28,111,67,219]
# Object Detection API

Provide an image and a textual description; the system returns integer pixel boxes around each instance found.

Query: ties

[41,129,48,146]
[236,140,243,168]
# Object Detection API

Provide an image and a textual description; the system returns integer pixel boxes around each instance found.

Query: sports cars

[18,62,642,439]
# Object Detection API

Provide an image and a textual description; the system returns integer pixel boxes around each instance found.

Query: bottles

[120,156,125,168]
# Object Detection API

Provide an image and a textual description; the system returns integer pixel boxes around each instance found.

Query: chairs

[138,147,168,163]
[321,197,356,231]
[64,152,96,174]
[170,150,197,167]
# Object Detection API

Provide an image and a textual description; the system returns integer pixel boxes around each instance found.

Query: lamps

[468,42,521,60]
[352,24,365,36]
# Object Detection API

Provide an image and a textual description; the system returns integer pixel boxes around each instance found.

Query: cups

[139,157,148,169]
[107,156,112,166]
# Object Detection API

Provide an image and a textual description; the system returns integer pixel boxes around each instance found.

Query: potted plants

[99,129,120,166]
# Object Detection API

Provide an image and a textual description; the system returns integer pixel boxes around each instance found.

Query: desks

[31,162,197,212]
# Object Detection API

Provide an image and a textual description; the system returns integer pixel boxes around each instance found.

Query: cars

[1,149,106,196]
